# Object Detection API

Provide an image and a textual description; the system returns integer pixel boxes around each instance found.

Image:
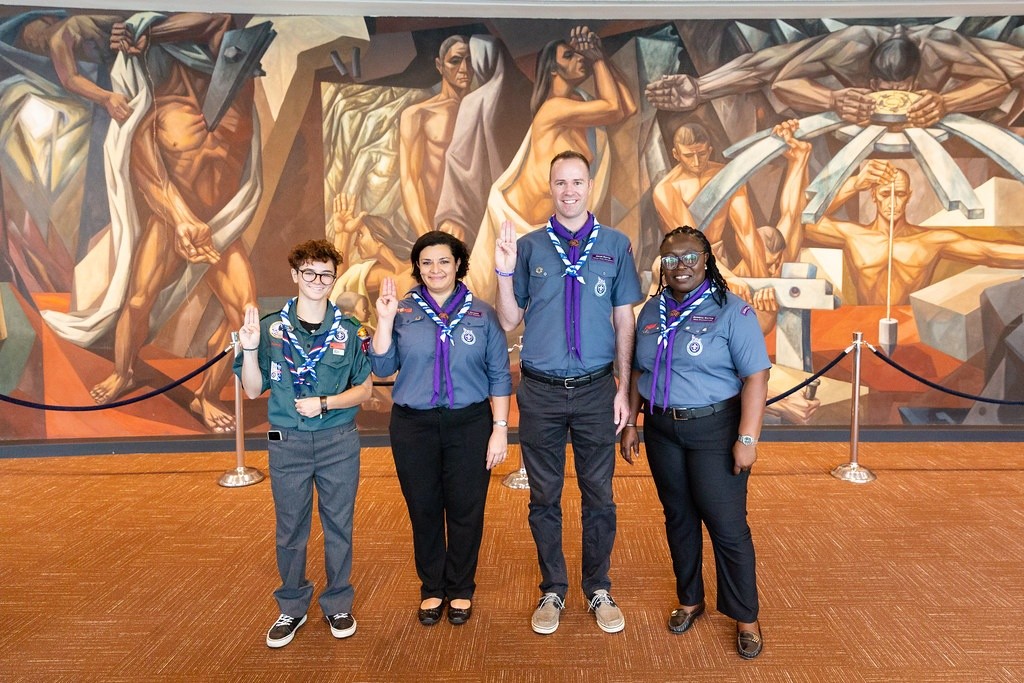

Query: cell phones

[267,430,283,441]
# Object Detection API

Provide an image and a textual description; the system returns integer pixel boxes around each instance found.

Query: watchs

[738,433,758,446]
[493,420,508,426]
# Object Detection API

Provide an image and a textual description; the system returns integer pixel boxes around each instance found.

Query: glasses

[661,250,705,270]
[295,267,337,285]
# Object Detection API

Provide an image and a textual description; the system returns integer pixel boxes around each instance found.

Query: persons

[620,226,772,657]
[370,231,512,624]
[241,239,372,648]
[495,150,642,631]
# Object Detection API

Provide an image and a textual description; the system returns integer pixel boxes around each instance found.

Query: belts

[522,361,612,389]
[644,391,741,421]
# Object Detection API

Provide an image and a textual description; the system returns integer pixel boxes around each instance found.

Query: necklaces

[296,315,325,333]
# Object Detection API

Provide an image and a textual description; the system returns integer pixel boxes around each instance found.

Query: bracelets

[320,395,328,414]
[242,348,259,351]
[626,423,637,427]
[495,268,515,277]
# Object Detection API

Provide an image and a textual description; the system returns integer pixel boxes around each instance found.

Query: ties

[657,281,717,349]
[280,296,342,384]
[283,325,329,399]
[421,281,467,408]
[650,279,711,415]
[411,289,473,347]
[546,214,600,285]
[553,210,593,357]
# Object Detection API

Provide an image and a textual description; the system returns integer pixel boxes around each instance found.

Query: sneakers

[587,589,625,633]
[531,593,565,634]
[266,611,307,647]
[326,612,356,638]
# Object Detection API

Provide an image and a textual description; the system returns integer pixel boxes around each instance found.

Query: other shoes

[737,620,763,659]
[418,599,445,624]
[448,599,472,624]
[668,600,705,634]
